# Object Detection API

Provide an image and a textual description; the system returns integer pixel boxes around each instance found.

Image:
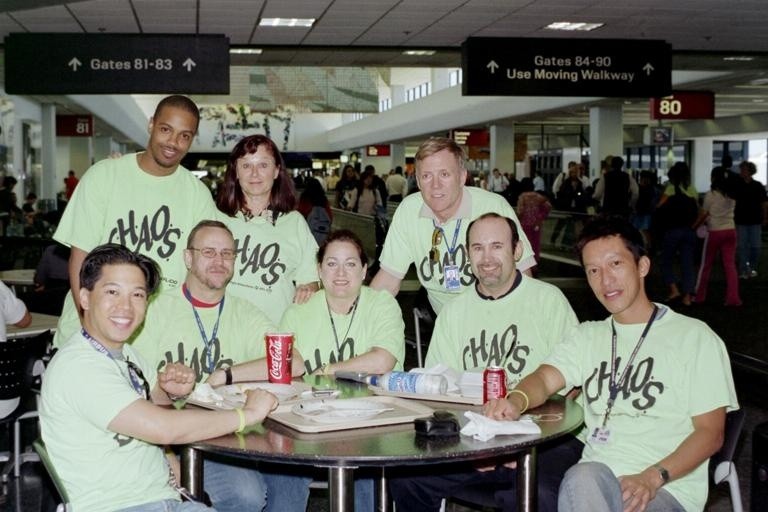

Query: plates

[291,400,386,423]
[300,389,342,403]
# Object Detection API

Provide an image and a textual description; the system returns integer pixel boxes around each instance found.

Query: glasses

[124,356,149,401]
[188,248,236,260]
[429,227,442,264]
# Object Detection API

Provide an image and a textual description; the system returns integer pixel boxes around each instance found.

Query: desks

[175,377,584,509]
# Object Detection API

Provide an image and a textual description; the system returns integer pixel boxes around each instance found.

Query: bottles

[366,370,447,399]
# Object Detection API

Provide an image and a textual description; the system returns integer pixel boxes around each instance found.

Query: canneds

[483,366,507,406]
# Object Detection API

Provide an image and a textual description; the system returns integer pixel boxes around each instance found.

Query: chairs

[0,328,52,481]
[712,401,749,512]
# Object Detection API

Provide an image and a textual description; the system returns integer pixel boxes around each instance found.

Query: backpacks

[306,206,331,246]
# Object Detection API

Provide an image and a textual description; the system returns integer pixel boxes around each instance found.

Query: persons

[279,135,767,512]
[0,169,79,442]
[38,95,333,512]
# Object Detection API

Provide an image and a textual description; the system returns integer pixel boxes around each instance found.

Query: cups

[264,330,297,386]
